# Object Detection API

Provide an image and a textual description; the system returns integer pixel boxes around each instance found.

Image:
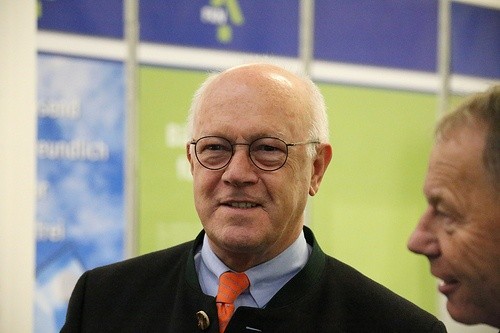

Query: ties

[215,271,249,333]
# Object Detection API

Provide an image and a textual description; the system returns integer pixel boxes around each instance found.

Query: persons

[60,63,448,333]
[407,85,500,333]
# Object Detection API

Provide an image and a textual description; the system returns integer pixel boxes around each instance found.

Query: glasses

[189,135,320,171]
